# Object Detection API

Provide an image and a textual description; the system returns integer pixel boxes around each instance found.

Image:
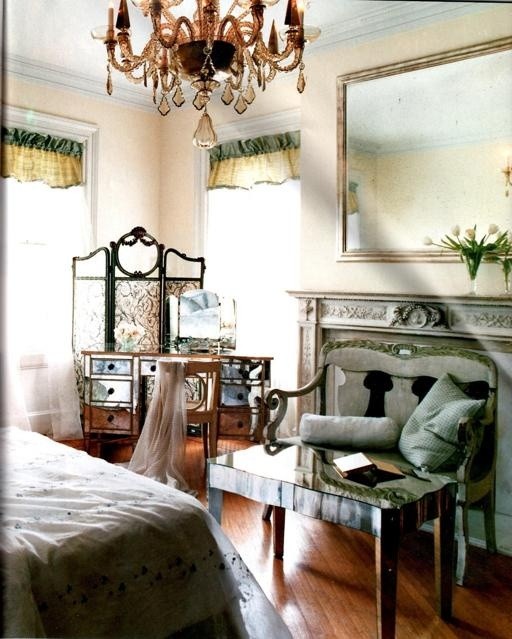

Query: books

[333,451,407,481]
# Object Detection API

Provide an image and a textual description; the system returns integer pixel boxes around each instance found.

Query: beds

[1,421,294,638]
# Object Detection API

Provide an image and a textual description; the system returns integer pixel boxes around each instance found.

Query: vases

[500,268,512,296]
[466,263,485,297]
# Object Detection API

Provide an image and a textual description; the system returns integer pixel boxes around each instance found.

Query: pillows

[299,412,399,451]
[397,372,486,473]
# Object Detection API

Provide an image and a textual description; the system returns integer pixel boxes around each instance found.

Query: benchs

[205,440,458,639]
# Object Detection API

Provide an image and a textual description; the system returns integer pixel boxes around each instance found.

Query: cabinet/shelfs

[80,350,274,443]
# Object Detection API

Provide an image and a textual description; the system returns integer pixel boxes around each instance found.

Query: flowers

[421,223,498,266]
[482,230,512,288]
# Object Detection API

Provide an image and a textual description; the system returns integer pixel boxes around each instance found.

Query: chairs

[146,359,221,480]
[260,339,499,587]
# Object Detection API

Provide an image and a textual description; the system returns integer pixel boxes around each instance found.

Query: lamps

[490,143,512,198]
[90,0,322,150]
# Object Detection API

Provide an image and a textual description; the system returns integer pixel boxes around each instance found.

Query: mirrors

[335,36,512,264]
